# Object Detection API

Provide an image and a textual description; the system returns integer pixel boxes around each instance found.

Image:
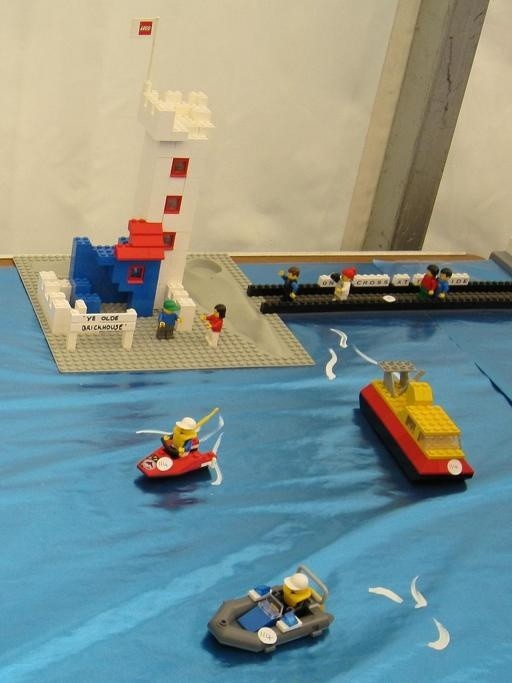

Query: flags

[129,14,159,40]
